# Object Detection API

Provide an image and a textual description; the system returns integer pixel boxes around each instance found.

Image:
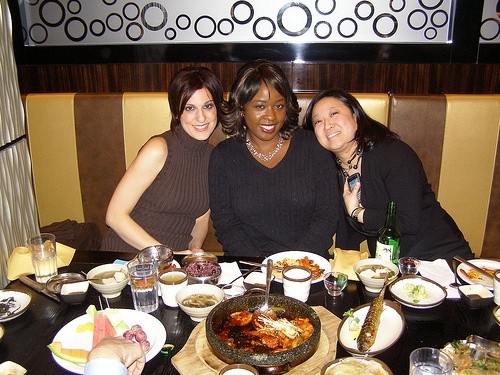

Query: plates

[457,258,500,291]
[261,251,332,282]
[52,309,167,374]
[0,287,32,322]
[124,258,181,285]
[46,273,85,293]
[441,306,500,375]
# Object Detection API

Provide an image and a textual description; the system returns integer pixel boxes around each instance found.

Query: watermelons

[91,314,117,348]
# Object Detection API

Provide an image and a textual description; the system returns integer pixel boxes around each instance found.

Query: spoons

[217,267,258,289]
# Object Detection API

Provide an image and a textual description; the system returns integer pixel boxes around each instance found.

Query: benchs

[21,92,500,258]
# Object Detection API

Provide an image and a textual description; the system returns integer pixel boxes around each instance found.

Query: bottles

[374,201,401,265]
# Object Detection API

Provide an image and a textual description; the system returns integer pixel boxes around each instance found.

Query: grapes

[122,325,150,352]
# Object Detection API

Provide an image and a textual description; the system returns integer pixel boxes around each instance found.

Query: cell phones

[347,173,362,202]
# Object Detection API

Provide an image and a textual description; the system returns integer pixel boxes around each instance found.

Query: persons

[301,89,476,260]
[99,66,224,254]
[208,60,341,261]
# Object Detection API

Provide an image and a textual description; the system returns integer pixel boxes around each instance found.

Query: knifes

[20,274,60,302]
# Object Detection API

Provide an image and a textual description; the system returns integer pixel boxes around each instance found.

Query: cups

[398,256,421,277]
[127,244,221,314]
[408,348,454,375]
[27,234,58,286]
[494,269,500,306]
[282,267,348,303]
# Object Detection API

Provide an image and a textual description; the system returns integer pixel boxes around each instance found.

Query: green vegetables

[343,308,360,324]
[449,339,498,373]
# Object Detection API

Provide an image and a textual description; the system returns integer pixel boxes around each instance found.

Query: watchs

[353,208,365,222]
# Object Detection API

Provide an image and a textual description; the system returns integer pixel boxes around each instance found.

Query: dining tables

[0,251,500,375]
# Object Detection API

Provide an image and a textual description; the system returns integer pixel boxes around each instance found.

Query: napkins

[329,247,369,282]
[6,240,77,282]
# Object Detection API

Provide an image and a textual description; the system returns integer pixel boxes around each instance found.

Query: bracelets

[351,207,359,217]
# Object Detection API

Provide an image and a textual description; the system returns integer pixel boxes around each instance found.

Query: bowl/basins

[320,300,407,375]
[87,264,130,299]
[243,272,275,290]
[176,284,224,322]
[353,258,494,309]
[206,287,321,375]
[60,282,90,304]
[218,364,260,375]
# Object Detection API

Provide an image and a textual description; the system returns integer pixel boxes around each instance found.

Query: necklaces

[246,131,284,162]
[336,145,362,169]
